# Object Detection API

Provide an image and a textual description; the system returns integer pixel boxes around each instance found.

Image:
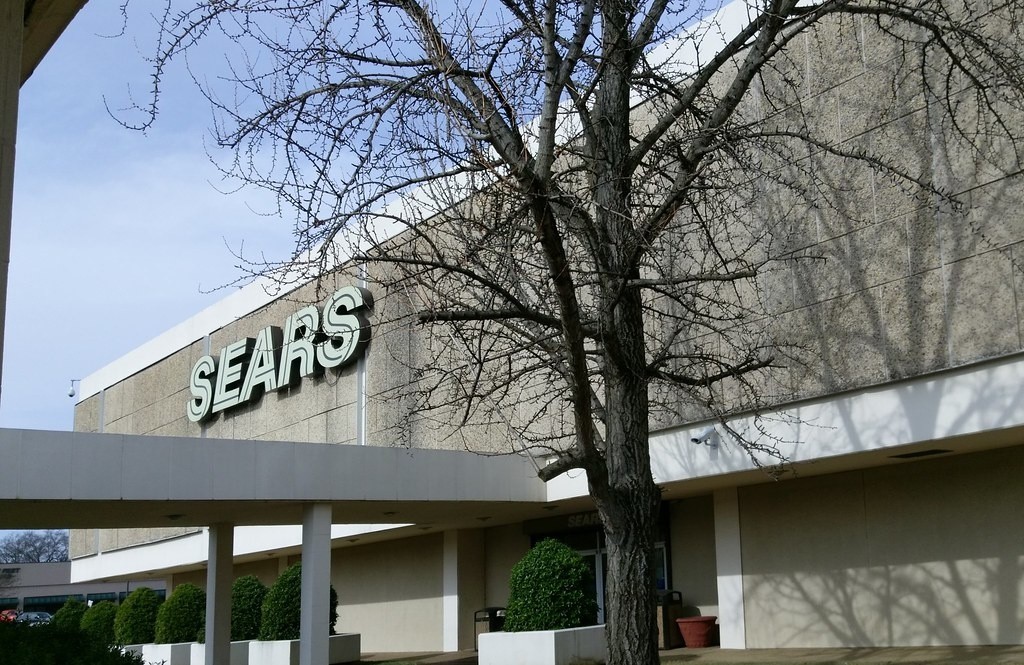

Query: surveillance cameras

[691,429,715,444]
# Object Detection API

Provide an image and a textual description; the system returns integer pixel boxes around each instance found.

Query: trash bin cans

[474,606,507,652]
[654,588,685,650]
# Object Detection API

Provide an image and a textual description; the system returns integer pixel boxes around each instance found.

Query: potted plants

[79,561,361,665]
[478,538,606,665]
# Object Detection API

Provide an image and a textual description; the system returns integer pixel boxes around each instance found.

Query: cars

[14,612,51,628]
[0,610,18,623]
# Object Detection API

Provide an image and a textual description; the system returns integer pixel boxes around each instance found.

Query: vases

[675,616,717,648]
